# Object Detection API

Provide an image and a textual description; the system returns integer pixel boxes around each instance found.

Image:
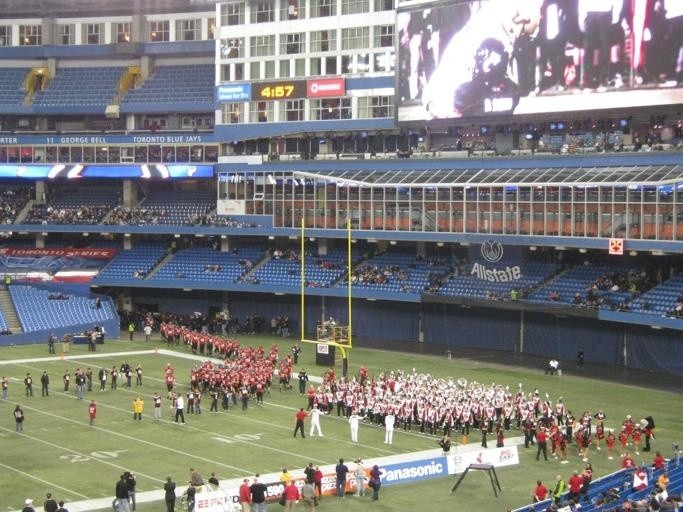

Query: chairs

[0,188,216,226]
[242,241,458,297]
[440,255,680,315]
[94,244,267,284]
[519,462,680,512]
[0,283,115,338]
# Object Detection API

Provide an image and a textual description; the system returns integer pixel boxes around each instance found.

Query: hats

[24,498,33,504]
